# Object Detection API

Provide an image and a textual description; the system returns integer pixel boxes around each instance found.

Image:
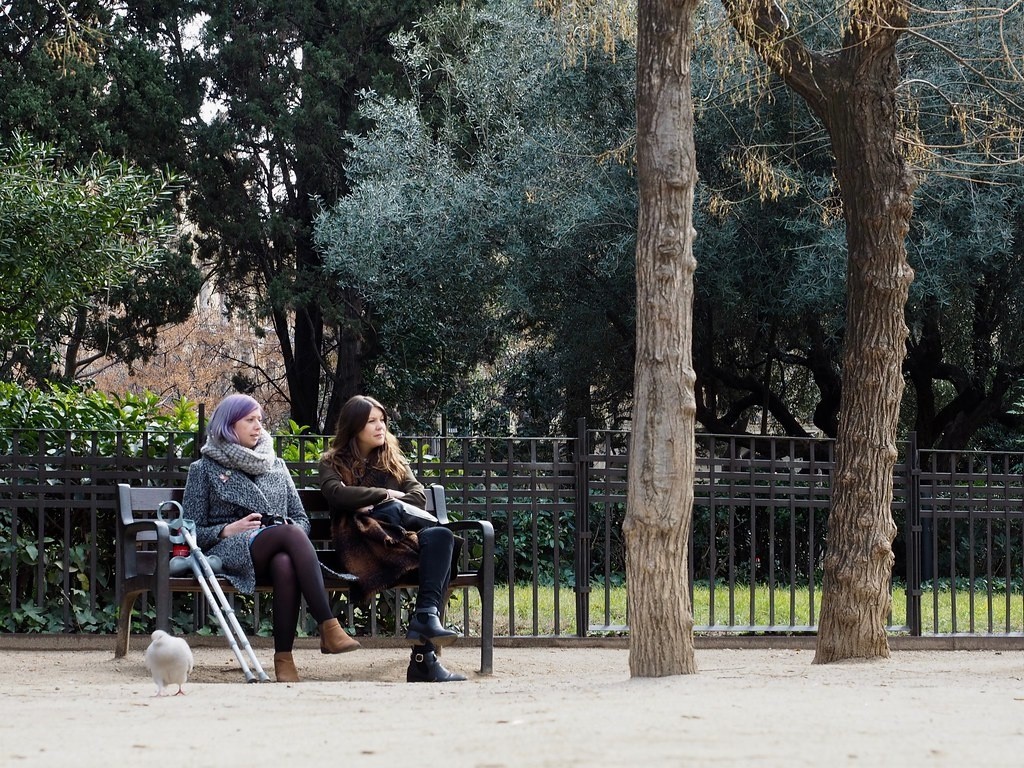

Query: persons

[180,392,361,682]
[317,395,468,682]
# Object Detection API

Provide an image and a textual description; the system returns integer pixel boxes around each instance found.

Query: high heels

[406,606,458,646]
[407,651,468,682]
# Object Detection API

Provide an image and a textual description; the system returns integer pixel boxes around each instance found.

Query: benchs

[114,483,495,677]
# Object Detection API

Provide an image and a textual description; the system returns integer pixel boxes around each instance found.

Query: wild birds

[145,629,194,697]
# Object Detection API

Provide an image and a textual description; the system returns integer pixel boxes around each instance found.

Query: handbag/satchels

[369,499,438,531]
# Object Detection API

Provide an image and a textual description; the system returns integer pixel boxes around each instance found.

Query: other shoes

[317,618,361,654]
[274,651,299,682]
